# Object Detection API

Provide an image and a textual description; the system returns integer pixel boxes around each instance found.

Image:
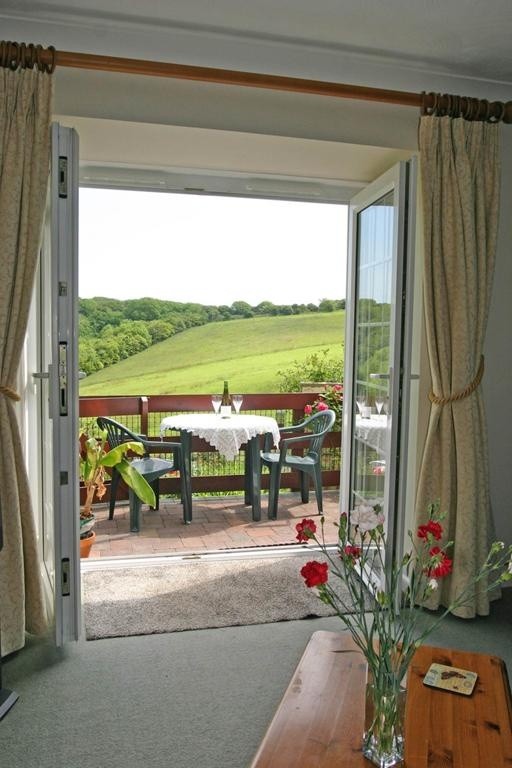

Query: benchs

[258,410,336,521]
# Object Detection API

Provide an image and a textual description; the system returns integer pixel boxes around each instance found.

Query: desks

[162,413,278,521]
[250,631,512,768]
[355,414,386,456]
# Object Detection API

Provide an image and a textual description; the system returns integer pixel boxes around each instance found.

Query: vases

[362,658,408,768]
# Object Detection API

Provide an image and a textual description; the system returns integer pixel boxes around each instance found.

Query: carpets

[81,550,415,640]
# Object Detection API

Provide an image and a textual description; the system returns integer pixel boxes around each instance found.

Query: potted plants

[79,422,157,557]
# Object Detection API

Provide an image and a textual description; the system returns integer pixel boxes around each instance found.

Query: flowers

[303,384,344,434]
[294,497,512,759]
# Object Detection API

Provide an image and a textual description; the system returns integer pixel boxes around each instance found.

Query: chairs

[94,415,191,534]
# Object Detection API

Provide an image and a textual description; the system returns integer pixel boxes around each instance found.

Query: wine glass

[375,397,385,415]
[232,395,243,415]
[211,394,222,415]
[356,397,367,413]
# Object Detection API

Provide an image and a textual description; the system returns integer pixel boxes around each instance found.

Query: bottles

[362,390,372,419]
[220,381,231,420]
